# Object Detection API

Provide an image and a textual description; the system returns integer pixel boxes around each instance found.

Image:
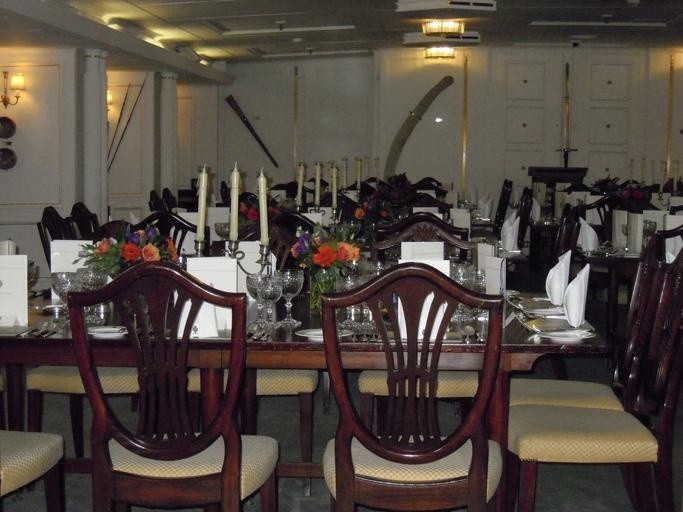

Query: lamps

[424,22,466,39]
[0,71,24,109]
[107,92,112,111]
[424,48,457,60]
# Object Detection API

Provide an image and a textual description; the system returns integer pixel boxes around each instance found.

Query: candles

[199,155,382,179]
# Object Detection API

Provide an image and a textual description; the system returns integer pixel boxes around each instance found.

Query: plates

[539,330,594,344]
[521,306,567,316]
[85,327,128,341]
[296,328,353,342]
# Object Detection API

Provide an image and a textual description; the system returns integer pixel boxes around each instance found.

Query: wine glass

[52,269,110,325]
[315,260,497,346]
[622,219,658,253]
[326,218,360,246]
[247,269,305,334]
